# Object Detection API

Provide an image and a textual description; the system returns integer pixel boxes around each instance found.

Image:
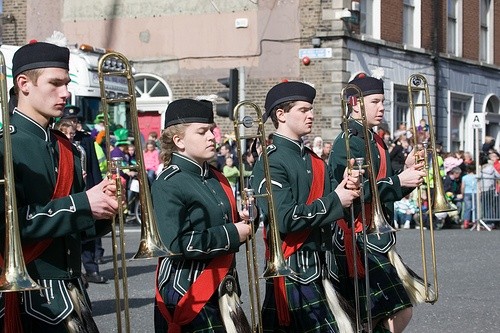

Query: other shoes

[423,226,427,230]
[394,219,398,228]
[415,226,421,229]
[464,220,468,228]
[404,220,410,229]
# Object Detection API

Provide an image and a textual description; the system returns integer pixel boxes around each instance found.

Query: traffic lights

[216,68,239,121]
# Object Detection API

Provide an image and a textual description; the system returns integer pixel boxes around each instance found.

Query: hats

[165,99,213,128]
[60,106,81,118]
[113,128,132,147]
[12,40,70,78]
[444,157,463,173]
[346,67,385,99]
[96,113,111,126]
[264,79,316,118]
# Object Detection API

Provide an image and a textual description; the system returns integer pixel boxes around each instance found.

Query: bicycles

[122,176,142,224]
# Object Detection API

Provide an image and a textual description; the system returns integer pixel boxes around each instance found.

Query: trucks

[2,43,136,127]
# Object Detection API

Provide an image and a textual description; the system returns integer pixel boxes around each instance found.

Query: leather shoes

[85,271,107,282]
[94,256,103,263]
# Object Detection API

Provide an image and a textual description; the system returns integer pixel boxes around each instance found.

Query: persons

[251,79,365,333]
[56,106,162,282]
[377,119,500,229]
[151,99,260,333]
[328,74,426,333]
[0,39,126,333]
[213,124,273,197]
[305,136,331,162]
